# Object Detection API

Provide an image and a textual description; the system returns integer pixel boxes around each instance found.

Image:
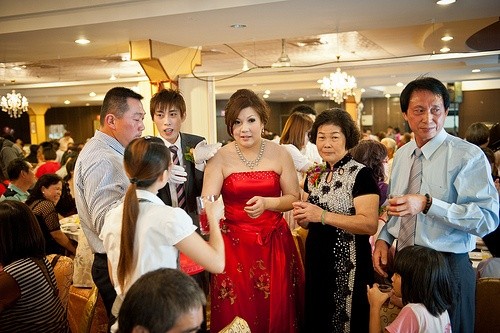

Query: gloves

[167,165,187,183]
[193,140,222,171]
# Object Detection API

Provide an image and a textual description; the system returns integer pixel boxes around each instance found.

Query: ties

[168,146,187,212]
[397,148,423,251]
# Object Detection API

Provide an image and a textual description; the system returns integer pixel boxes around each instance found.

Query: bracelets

[423,193,430,214]
[322,210,327,225]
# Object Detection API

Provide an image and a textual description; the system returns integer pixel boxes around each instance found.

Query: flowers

[182,146,195,163]
[306,161,327,188]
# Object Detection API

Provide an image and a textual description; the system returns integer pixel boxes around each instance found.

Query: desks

[59,215,79,242]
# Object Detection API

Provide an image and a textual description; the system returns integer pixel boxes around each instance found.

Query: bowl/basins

[68,223,78,232]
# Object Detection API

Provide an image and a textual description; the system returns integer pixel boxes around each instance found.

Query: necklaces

[236,139,265,169]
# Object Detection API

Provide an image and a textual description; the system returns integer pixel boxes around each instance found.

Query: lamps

[0,79,29,118]
[318,55,357,104]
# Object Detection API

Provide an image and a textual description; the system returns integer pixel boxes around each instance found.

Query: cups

[388,193,404,212]
[196,194,224,235]
[291,200,310,211]
[378,285,392,307]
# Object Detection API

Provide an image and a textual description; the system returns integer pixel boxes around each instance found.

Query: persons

[119,269,208,333]
[201,89,302,333]
[150,89,223,238]
[367,246,452,333]
[0,133,85,178]
[280,110,317,230]
[55,158,77,218]
[476,225,500,277]
[372,77,500,333]
[0,200,70,333]
[26,174,78,259]
[290,104,323,164]
[291,108,380,333]
[352,127,411,207]
[0,160,34,204]
[466,123,500,191]
[100,136,224,303]
[74,87,147,333]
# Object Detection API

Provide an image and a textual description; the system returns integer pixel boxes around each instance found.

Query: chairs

[46,254,99,333]
[476,276,500,332]
